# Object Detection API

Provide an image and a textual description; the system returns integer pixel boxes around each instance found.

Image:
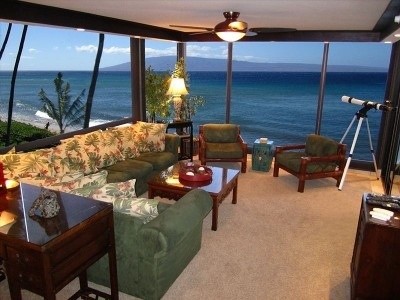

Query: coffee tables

[146,159,241,231]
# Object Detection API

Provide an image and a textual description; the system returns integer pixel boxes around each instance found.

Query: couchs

[0,117,213,300]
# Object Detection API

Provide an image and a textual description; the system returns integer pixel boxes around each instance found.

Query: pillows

[0,121,166,224]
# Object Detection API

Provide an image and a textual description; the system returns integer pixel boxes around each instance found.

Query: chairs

[273,134,347,193]
[199,123,247,173]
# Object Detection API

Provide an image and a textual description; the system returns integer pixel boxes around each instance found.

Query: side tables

[252,139,274,172]
[156,120,193,162]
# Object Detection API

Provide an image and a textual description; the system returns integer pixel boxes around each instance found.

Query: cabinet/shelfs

[349,192,400,300]
[0,182,120,300]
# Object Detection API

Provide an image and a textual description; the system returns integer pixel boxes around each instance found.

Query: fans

[169,12,296,42]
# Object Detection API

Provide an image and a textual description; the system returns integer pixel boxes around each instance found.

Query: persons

[44,122,50,129]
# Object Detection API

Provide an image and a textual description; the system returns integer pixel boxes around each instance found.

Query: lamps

[214,12,248,42]
[165,78,189,122]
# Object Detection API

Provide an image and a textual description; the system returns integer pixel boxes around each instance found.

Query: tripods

[338,106,380,191]
